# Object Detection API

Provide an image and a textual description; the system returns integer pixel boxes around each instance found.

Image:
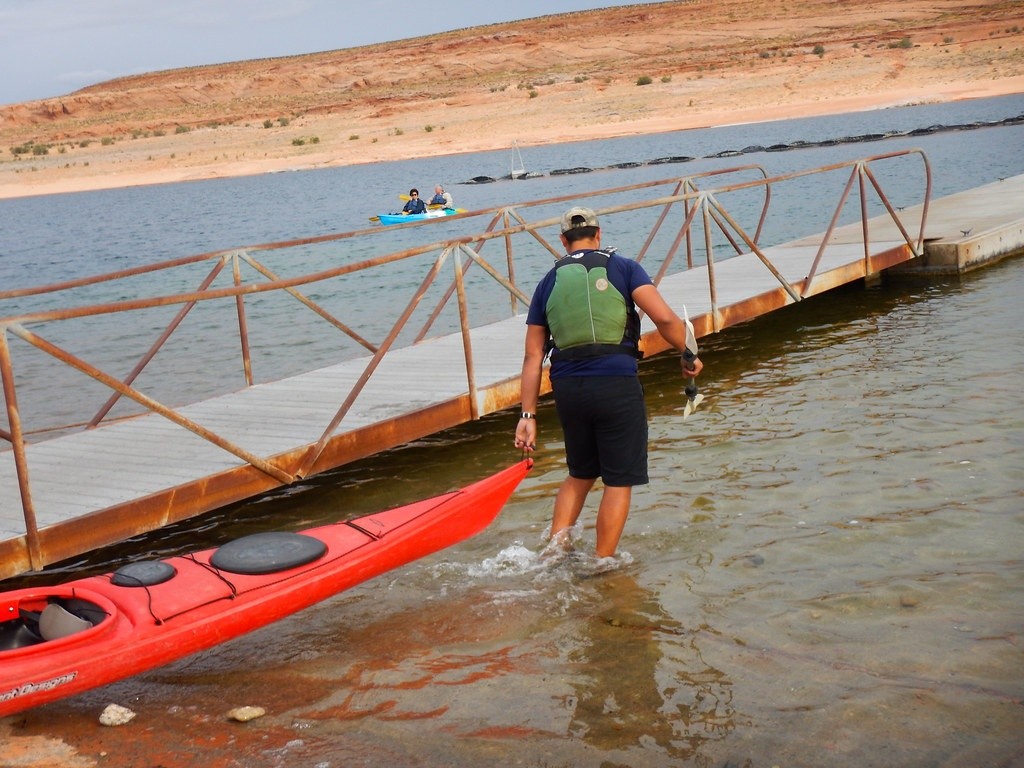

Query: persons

[513,205,704,560]
[426,183,453,210]
[402,188,424,214]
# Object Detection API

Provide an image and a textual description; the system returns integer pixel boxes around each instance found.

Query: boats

[377,207,469,228]
[1,458,534,718]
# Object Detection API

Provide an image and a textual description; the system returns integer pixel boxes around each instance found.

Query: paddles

[399,194,468,214]
[368,204,443,222]
[681,304,706,422]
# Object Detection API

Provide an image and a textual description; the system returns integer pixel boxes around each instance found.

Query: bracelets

[519,412,537,419]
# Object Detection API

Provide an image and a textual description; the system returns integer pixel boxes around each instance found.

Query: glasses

[411,193,417,196]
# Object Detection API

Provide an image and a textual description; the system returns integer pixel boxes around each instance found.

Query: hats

[560,206,600,234]
